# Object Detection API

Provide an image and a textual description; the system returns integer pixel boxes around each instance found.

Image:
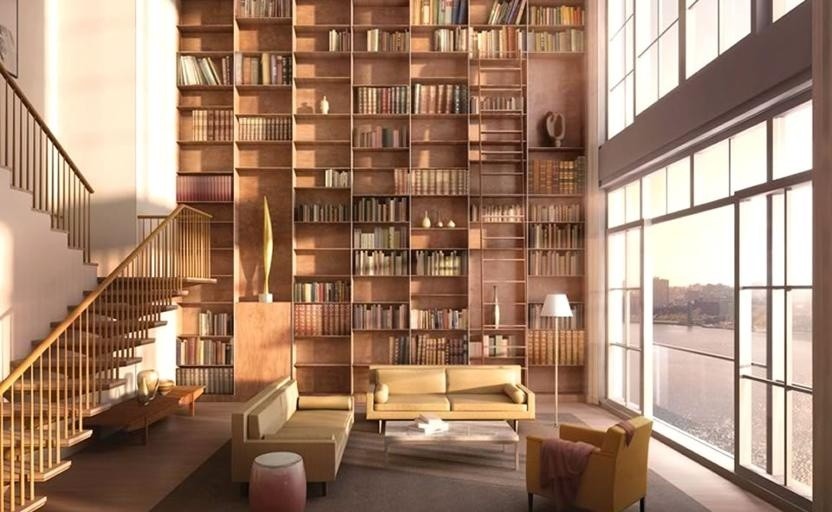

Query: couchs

[365,364,537,435]
[231,374,355,498]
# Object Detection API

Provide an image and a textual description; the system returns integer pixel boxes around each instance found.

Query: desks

[74,385,206,446]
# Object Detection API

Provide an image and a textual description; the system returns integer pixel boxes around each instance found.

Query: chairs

[525,416,654,512]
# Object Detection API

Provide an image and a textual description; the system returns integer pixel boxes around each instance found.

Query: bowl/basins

[157,381,173,396]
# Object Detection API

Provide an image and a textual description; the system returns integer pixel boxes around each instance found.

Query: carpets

[146,411,712,512]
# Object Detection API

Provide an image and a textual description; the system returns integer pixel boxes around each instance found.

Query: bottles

[320,95,330,115]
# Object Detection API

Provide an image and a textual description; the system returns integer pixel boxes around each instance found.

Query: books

[177,56,233,85]
[353,125,408,148]
[353,0,527,115]
[471,203,522,222]
[528,4,586,52]
[293,281,351,335]
[177,312,235,394]
[394,169,467,195]
[469,335,516,357]
[353,304,409,329]
[416,250,468,276]
[325,169,350,188]
[528,304,586,365]
[353,196,408,276]
[236,1,293,141]
[529,157,584,194]
[177,175,232,201]
[329,27,352,51]
[192,109,234,141]
[529,204,585,275]
[411,307,467,365]
[388,334,409,364]
[298,203,350,222]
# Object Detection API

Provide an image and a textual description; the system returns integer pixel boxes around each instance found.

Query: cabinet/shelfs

[171,0,592,402]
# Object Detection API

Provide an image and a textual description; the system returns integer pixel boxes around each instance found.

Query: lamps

[539,292,574,425]
